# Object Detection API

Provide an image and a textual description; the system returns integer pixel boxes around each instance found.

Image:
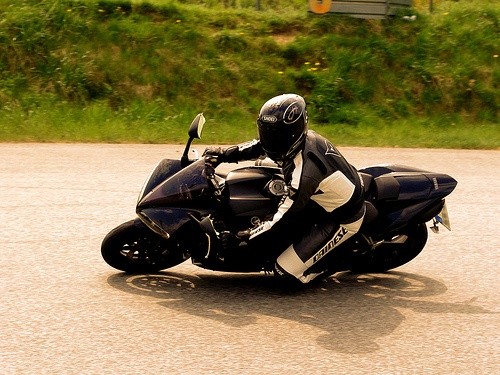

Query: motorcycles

[101,112,458,275]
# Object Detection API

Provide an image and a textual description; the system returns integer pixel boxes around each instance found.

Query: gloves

[217,231,250,256]
[202,146,238,164]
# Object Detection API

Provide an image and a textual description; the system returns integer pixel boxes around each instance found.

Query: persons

[202,93,367,293]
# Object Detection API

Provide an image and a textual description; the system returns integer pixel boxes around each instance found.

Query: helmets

[257,94,308,161]
